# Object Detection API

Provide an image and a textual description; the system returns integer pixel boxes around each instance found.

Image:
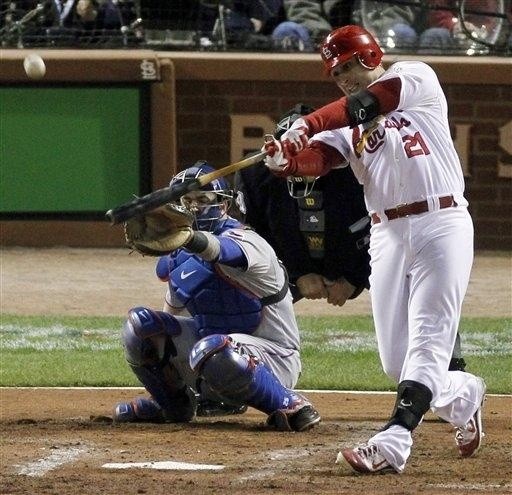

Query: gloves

[260,134,291,175]
[295,273,356,306]
[280,116,310,158]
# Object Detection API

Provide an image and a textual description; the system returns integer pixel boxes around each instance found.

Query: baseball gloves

[125,193,195,255]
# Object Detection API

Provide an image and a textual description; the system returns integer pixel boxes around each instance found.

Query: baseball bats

[106,146,275,226]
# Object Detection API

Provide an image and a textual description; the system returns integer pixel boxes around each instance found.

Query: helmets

[320,25,384,75]
[168,160,238,234]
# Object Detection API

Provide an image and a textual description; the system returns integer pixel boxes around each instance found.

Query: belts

[372,196,457,224]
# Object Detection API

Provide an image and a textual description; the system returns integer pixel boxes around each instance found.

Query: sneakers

[454,376,487,458]
[112,400,166,423]
[195,393,248,417]
[290,407,322,432]
[335,443,398,474]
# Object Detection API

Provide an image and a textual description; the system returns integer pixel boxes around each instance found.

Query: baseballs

[24,54,47,79]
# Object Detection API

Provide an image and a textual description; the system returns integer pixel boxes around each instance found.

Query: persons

[113,159,321,432]
[1,0,512,55]
[260,25,486,475]
[228,103,467,371]
